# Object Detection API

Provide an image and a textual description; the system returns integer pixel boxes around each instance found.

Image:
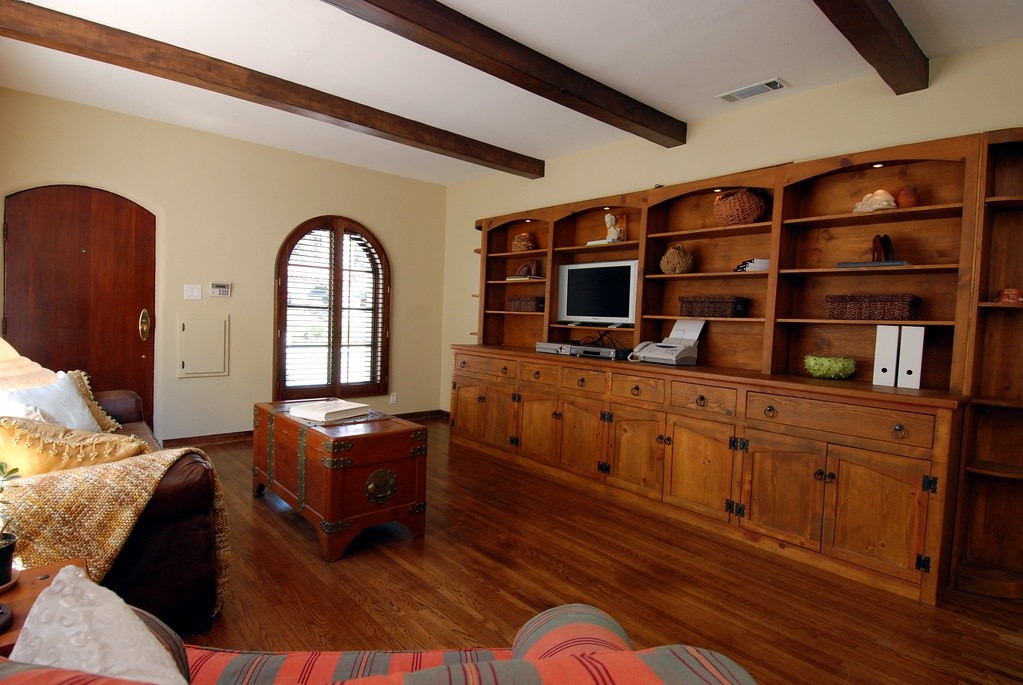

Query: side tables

[0,557,92,658]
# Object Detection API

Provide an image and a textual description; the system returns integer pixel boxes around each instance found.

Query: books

[586,239,618,246]
[290,401,370,421]
[506,275,544,280]
[733,258,769,271]
[835,260,910,268]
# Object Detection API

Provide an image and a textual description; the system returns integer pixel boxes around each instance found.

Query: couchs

[0,603,759,685]
[0,390,220,635]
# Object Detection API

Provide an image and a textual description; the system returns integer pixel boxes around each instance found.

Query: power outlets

[390,392,397,404]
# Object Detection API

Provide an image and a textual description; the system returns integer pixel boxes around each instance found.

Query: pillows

[8,568,188,685]
[68,369,123,433]
[0,371,102,433]
[0,416,152,476]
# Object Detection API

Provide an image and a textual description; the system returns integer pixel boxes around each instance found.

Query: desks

[250,397,428,562]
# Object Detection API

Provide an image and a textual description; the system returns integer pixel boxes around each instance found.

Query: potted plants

[0,461,22,593]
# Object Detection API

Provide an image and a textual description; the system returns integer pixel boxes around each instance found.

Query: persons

[605,214,619,239]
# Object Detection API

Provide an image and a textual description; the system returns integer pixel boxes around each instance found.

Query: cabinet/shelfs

[447,133,983,608]
[948,128,1023,602]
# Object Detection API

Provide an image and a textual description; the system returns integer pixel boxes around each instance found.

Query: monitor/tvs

[558,260,638,328]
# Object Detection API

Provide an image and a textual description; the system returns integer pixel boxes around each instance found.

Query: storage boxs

[678,295,752,318]
[825,292,921,320]
